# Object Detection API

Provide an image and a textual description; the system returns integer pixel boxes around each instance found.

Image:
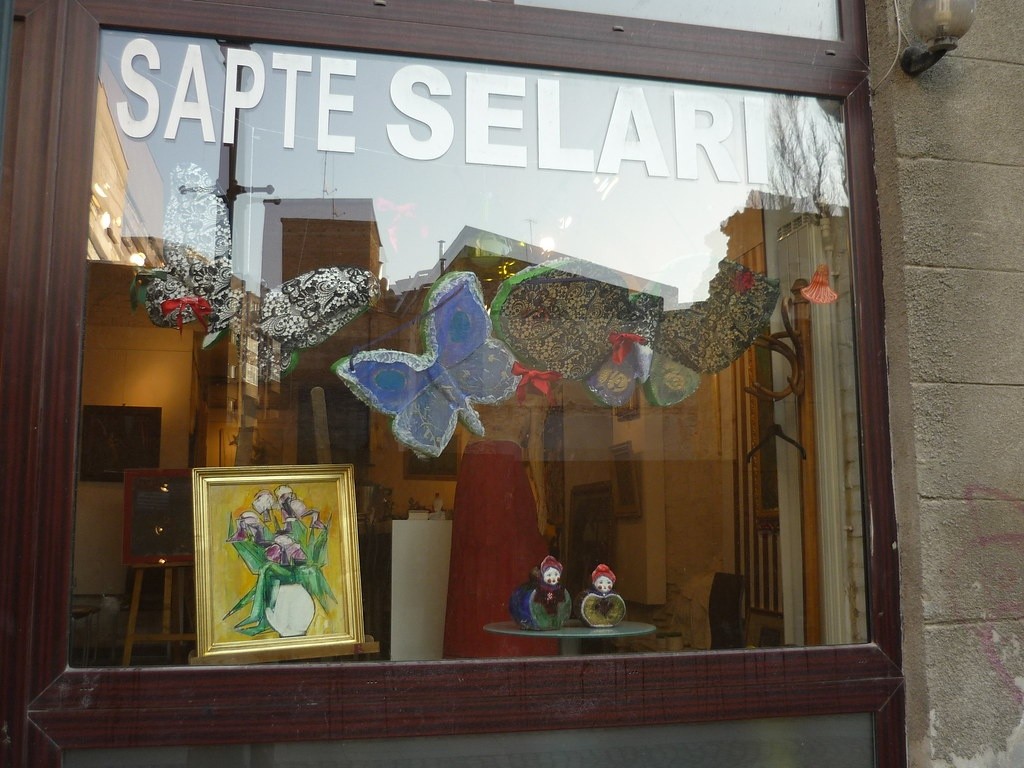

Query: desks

[483,619,660,655]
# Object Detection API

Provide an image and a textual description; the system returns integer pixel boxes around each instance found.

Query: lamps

[900,0,977,77]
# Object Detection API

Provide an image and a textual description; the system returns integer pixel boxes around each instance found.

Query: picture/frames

[402,433,461,481]
[565,480,615,618]
[192,460,369,661]
[78,405,163,483]
[608,441,640,518]
[123,466,191,566]
[614,387,639,422]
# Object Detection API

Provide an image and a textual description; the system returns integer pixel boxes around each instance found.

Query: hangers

[746,397,809,466]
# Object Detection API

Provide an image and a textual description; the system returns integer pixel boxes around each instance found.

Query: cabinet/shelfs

[375,516,451,661]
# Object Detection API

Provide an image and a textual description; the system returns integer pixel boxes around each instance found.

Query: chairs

[703,571,749,651]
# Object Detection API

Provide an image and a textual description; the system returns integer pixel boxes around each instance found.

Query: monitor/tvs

[81,404,162,483]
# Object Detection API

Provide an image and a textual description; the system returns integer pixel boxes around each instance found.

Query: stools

[71,600,101,666]
